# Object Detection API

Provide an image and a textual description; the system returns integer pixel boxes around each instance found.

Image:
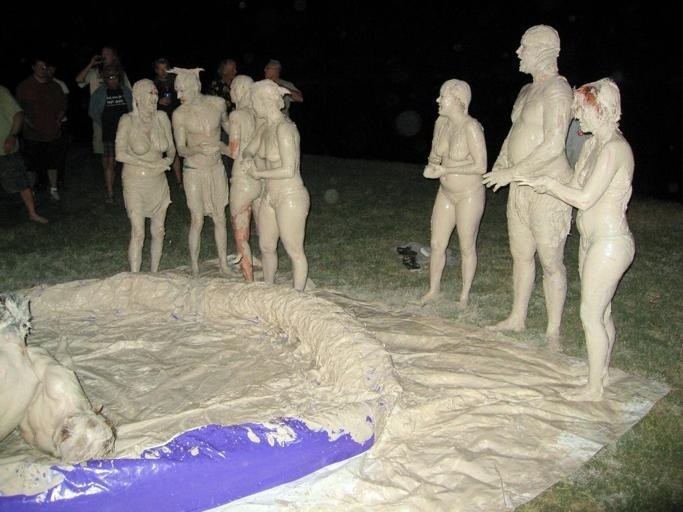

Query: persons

[513,78,636,402]
[1,324,116,465]
[1,59,70,224]
[115,56,310,293]
[76,48,135,203]
[482,24,573,353]
[418,79,488,311]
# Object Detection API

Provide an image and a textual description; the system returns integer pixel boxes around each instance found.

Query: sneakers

[51,188,60,202]
[103,192,114,203]
[29,216,48,225]
